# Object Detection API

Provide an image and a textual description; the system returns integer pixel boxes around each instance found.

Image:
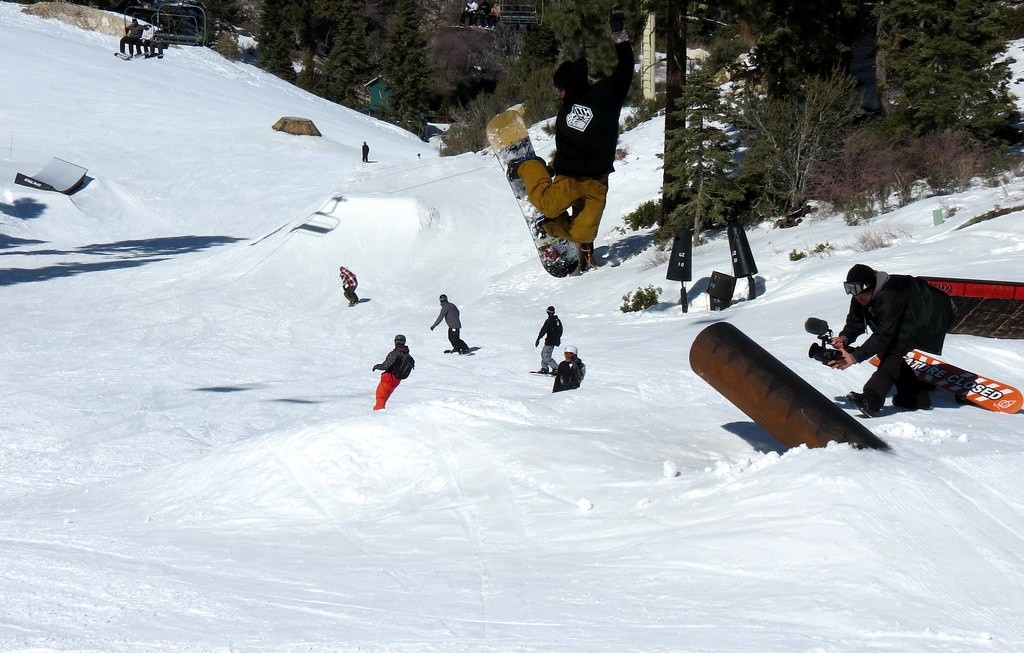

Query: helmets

[395,335,406,345]
[564,345,578,354]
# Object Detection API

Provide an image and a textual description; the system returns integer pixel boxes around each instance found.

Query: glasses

[843,282,863,296]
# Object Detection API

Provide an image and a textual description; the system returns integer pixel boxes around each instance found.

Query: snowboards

[688,321,893,454]
[486,110,580,278]
[867,349,1024,413]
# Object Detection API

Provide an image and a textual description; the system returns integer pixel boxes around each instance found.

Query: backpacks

[389,350,415,379]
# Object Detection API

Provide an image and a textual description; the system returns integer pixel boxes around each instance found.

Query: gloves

[609,9,624,32]
[373,365,378,371]
[536,339,539,347]
[431,326,434,331]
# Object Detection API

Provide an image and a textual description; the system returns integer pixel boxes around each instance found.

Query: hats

[547,306,555,313]
[847,264,877,296]
[440,295,447,301]
[553,61,572,91]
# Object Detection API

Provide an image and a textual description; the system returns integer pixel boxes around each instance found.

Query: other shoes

[892,394,933,410]
[461,348,470,354]
[539,368,549,374]
[509,156,546,180]
[552,368,559,375]
[847,392,874,417]
[535,210,569,232]
[452,347,460,352]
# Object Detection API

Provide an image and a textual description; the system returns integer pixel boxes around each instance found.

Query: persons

[119,18,168,59]
[430,294,469,353]
[372,335,409,410]
[361,141,369,162]
[339,266,359,306]
[826,263,958,417]
[552,346,586,392]
[534,305,564,375]
[504,8,635,244]
[457,0,501,30]
